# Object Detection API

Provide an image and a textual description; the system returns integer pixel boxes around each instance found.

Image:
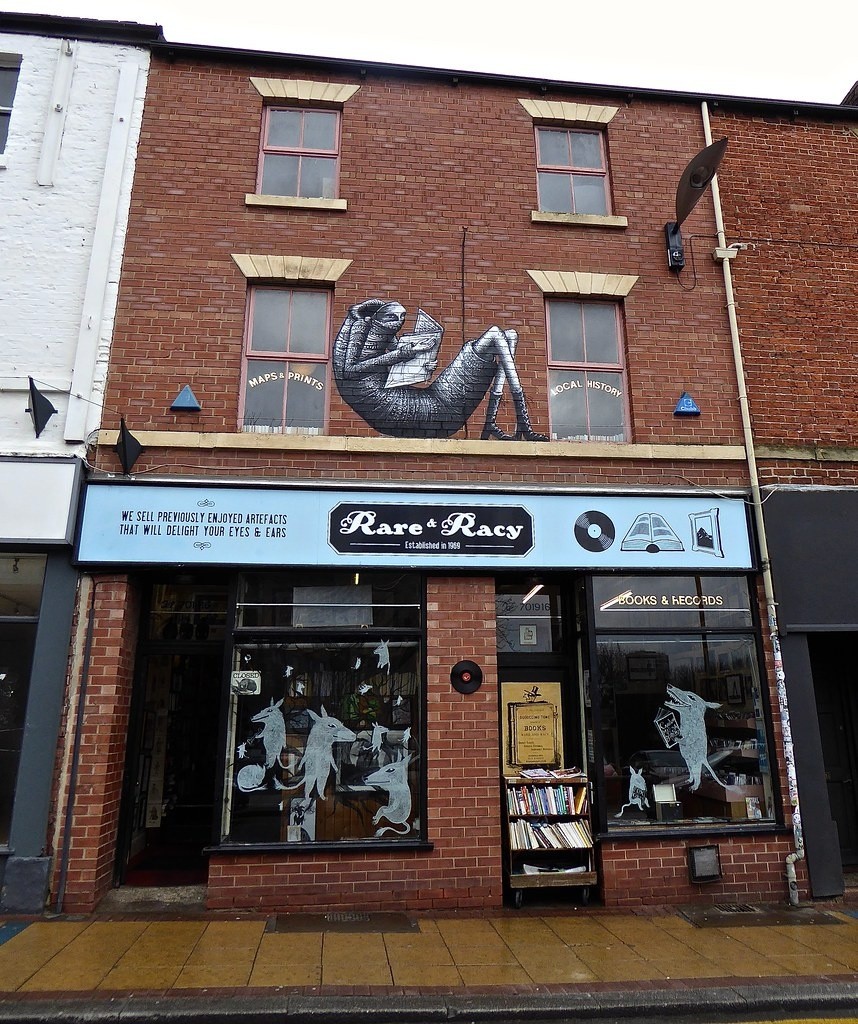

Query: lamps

[353,572,360,586]
[13,559,19,573]
[661,137,730,274]
[599,590,634,610]
[113,417,145,477]
[289,363,318,378]
[23,376,57,441]
[521,584,545,605]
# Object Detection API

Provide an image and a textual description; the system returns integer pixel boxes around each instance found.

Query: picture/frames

[627,593,753,706]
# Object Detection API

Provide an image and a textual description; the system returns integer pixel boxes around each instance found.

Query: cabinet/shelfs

[688,783,766,818]
[503,775,599,889]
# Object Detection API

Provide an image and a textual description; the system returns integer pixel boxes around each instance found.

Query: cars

[648,746,763,816]
[624,748,688,778]
[233,750,284,812]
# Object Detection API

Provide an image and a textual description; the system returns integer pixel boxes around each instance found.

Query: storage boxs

[705,718,724,728]
[653,783,684,822]
[726,718,756,729]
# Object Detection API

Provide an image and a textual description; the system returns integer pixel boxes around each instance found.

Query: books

[705,739,759,750]
[502,766,595,850]
[745,796,763,819]
[282,733,417,785]
[713,711,756,721]
[283,671,417,697]
[286,699,411,728]
[702,770,761,786]
